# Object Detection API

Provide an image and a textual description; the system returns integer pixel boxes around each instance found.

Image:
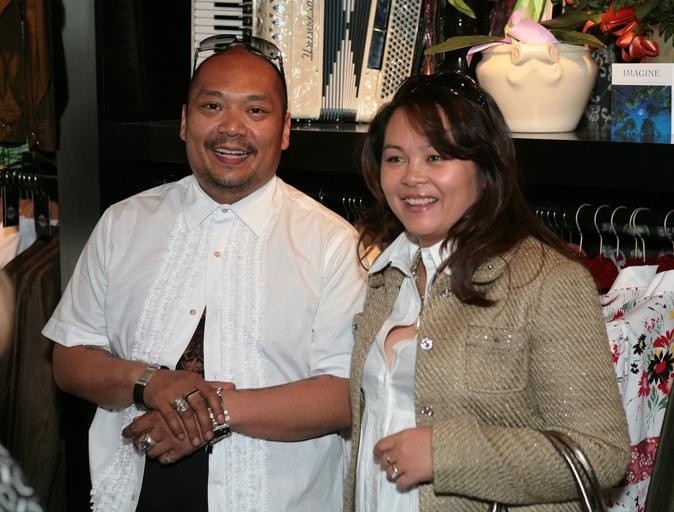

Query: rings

[171,387,201,412]
[164,454,176,465]
[137,431,156,452]
[382,452,401,482]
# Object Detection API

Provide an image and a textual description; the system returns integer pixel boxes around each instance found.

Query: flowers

[421,1,662,67]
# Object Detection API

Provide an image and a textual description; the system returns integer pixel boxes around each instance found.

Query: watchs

[206,386,232,445]
[130,361,169,411]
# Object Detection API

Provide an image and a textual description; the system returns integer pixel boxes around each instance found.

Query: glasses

[193,33,286,94]
[392,71,494,126]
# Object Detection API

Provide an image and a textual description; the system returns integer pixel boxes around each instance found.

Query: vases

[477,44,598,135]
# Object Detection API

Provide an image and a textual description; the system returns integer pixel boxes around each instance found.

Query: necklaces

[410,248,426,331]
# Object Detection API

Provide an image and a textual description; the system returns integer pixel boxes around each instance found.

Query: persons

[51,46,366,512]
[348,73,632,512]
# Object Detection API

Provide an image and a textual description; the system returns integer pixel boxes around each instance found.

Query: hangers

[2,169,59,221]
[573,201,674,272]
